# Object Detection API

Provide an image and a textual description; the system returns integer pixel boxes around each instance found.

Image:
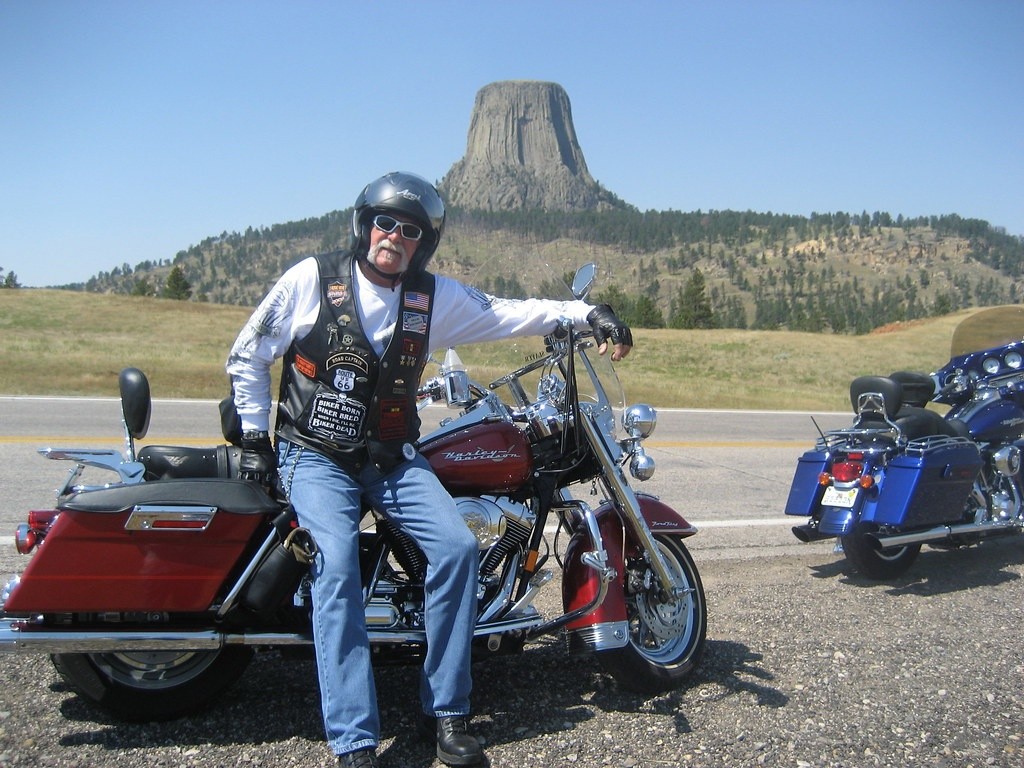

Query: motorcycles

[2,263,711,723]
[784,339,1024,580]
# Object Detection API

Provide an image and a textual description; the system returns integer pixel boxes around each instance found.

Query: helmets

[351,171,446,279]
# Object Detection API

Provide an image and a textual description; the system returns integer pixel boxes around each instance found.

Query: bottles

[443,344,467,375]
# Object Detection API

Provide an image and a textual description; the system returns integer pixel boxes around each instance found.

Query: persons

[225,172,633,768]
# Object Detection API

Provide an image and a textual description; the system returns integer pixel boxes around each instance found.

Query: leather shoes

[339,746,378,768]
[434,716,481,765]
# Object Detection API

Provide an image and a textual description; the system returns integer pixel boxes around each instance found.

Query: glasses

[367,214,427,241]
